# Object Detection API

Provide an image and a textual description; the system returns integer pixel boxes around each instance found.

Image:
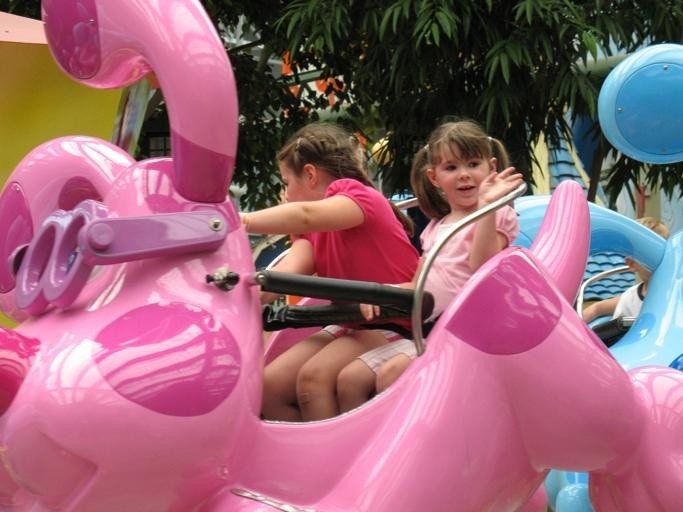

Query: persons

[578,216,670,331]
[334,111,525,416]
[234,121,421,424]
